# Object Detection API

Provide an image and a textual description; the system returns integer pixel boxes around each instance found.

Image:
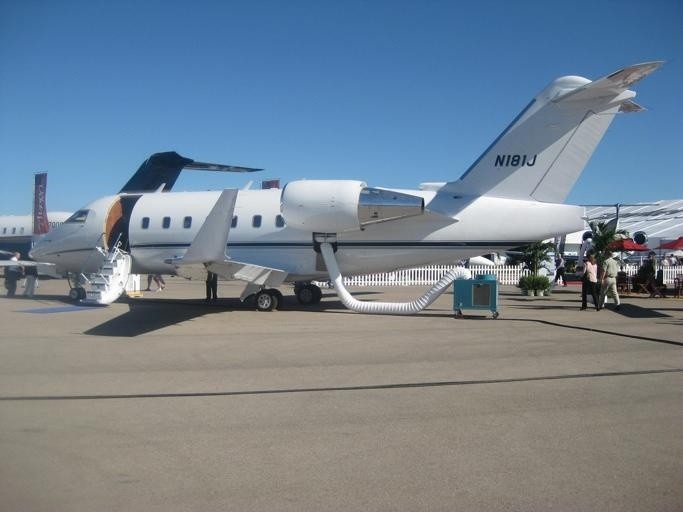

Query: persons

[145,273,152,291]
[6,251,20,297]
[22,255,39,296]
[154,272,163,291]
[597,250,622,311]
[616,251,682,295]
[580,254,600,312]
[553,253,567,287]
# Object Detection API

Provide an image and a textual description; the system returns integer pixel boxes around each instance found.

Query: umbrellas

[606,239,650,271]
[654,234,683,249]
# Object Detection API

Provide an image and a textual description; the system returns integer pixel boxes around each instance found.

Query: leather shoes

[580,307,587,310]
[615,305,622,310]
[597,306,600,311]
[600,306,605,309]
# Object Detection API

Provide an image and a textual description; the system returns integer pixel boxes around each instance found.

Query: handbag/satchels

[581,272,588,282]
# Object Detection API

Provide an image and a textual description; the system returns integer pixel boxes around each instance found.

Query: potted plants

[507,242,553,298]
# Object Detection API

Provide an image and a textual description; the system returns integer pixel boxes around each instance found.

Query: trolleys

[453,274,503,319]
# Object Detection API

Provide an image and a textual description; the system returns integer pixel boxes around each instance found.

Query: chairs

[615,273,682,299]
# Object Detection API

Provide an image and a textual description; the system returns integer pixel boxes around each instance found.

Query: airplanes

[0,211,75,279]
[27,60,669,311]
